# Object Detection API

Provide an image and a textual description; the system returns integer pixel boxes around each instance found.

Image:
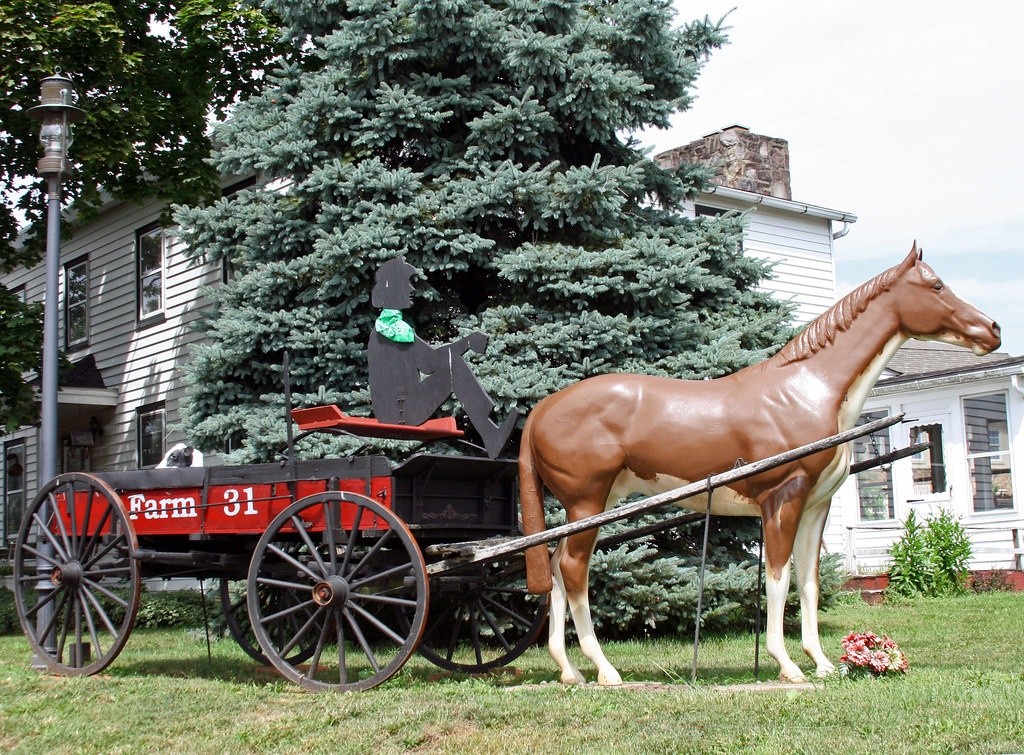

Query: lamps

[89,416,104,441]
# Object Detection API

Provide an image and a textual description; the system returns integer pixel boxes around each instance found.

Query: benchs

[290,404,465,440]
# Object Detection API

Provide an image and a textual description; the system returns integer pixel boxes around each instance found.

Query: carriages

[10,239,1002,697]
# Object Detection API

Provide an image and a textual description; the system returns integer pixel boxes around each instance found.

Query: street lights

[21,65,89,672]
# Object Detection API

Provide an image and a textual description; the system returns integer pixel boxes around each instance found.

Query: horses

[516,240,1003,684]
